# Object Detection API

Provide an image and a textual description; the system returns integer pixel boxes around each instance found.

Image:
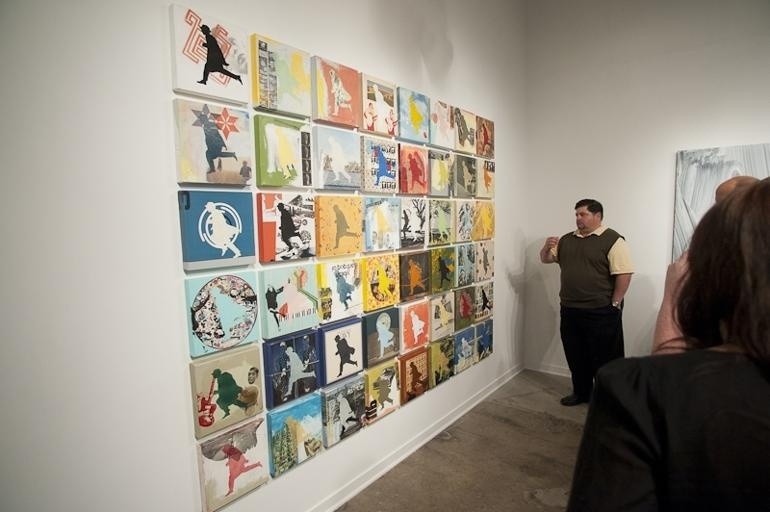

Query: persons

[563,176,769,511]
[247,366,260,383]
[649,251,690,355]
[538,199,634,406]
[714,176,759,203]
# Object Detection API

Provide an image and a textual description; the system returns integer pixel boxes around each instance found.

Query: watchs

[611,301,620,311]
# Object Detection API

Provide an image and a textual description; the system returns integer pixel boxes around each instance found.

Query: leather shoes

[558,392,589,408]
